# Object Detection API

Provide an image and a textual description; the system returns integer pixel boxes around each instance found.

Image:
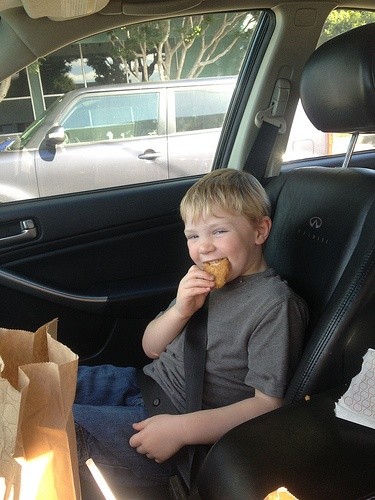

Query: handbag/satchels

[0,318,83,500]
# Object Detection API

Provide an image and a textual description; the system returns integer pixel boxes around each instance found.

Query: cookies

[203,258,231,288]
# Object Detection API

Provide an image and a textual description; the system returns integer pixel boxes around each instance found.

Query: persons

[64,168,310,485]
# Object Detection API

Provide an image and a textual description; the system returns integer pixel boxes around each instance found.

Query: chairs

[78,21,375,500]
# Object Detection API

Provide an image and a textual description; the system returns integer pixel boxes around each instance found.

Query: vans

[0,75,334,206]
